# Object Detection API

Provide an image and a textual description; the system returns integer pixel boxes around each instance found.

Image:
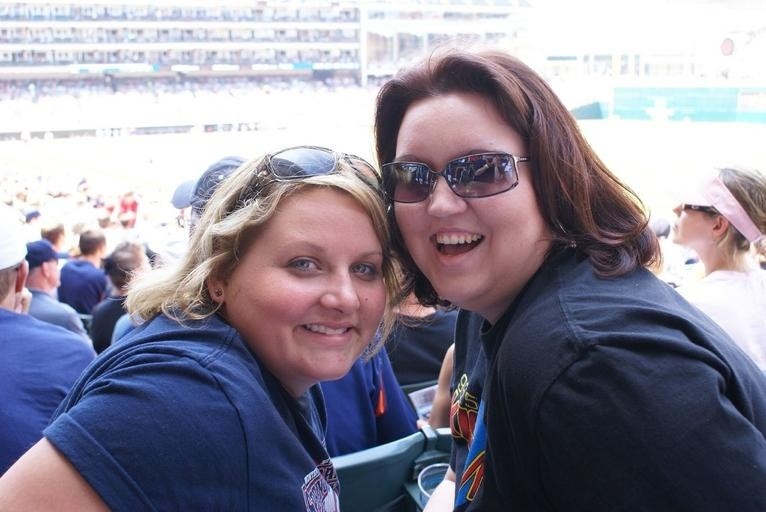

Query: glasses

[379,152,528,203]
[234,146,382,212]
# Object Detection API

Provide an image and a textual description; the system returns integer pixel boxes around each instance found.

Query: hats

[173,156,246,216]
[680,178,764,244]
[0,202,27,270]
[27,240,69,267]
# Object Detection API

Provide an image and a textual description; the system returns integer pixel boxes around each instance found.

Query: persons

[651,165,765,374]
[1,168,160,473]
[368,50,766,509]
[174,154,458,457]
[0,140,397,512]
